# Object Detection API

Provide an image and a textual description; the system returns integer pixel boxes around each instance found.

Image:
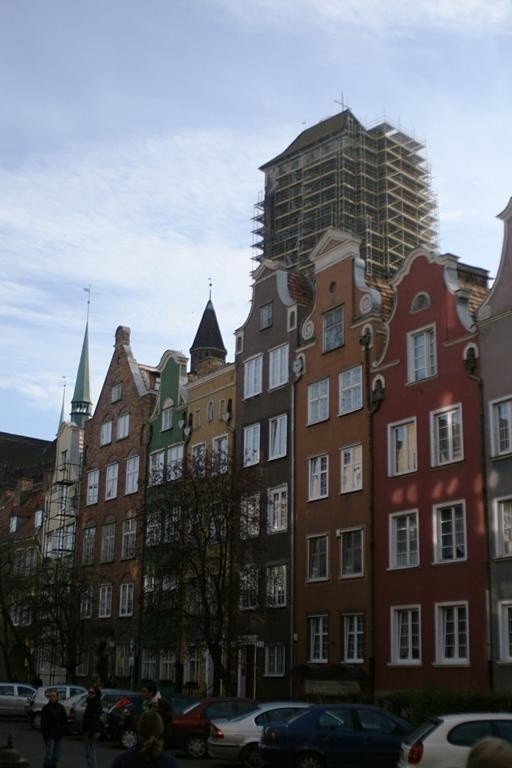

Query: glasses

[89,691,95,695]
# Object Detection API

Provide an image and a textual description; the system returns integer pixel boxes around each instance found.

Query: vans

[396,711,512,768]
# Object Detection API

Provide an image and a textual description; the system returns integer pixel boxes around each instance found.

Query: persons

[465,737,512,768]
[41,687,68,768]
[82,671,183,768]
[34,673,43,689]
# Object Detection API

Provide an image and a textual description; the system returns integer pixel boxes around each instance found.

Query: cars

[1,682,198,751]
[256,703,415,767]
[166,696,264,761]
[207,700,380,767]
